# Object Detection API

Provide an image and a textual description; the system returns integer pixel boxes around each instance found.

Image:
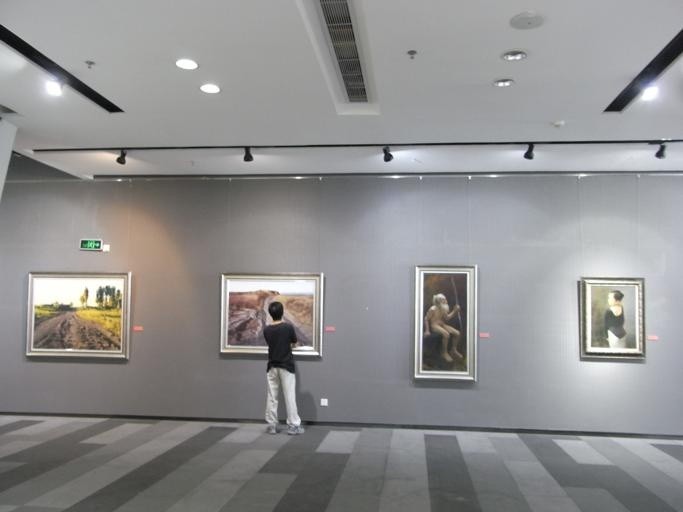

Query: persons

[423,293,463,361]
[604,291,626,348]
[263,302,304,435]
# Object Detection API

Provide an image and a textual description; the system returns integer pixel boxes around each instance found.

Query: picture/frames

[217,270,324,359]
[576,275,646,362]
[412,264,479,385]
[23,269,132,360]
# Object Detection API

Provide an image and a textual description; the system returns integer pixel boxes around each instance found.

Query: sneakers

[267,424,277,433]
[288,426,304,434]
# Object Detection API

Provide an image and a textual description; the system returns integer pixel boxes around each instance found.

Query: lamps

[113,139,670,167]
